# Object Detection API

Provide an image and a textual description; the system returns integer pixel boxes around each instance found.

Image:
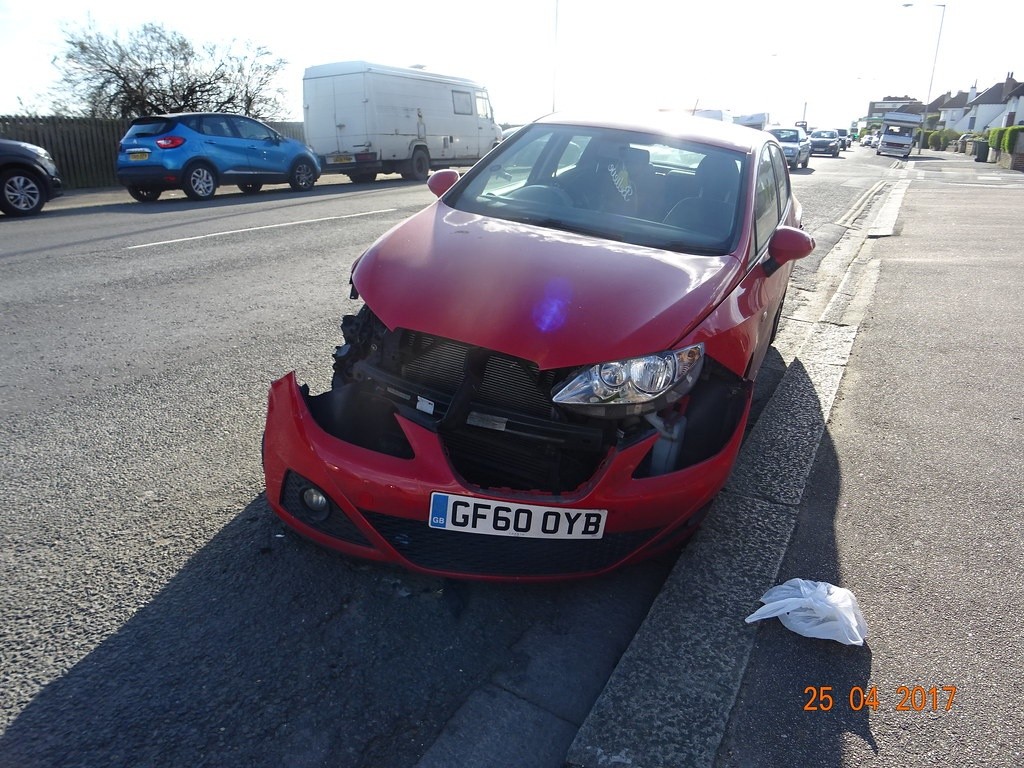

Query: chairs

[663,155,740,238]
[889,129,893,134]
[192,125,205,133]
[210,124,225,135]
[550,139,639,217]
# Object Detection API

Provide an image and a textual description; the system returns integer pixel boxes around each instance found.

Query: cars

[260,99,817,586]
[116,111,321,203]
[0,137,65,220]
[676,100,880,173]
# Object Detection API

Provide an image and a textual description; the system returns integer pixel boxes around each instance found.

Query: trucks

[303,64,504,184]
[877,112,923,160]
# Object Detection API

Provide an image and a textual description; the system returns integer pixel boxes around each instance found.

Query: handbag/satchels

[745,577,867,646]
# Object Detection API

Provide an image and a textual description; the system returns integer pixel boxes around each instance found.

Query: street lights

[902,3,945,155]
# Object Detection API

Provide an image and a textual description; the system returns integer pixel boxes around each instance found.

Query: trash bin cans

[975,141,988,162]
